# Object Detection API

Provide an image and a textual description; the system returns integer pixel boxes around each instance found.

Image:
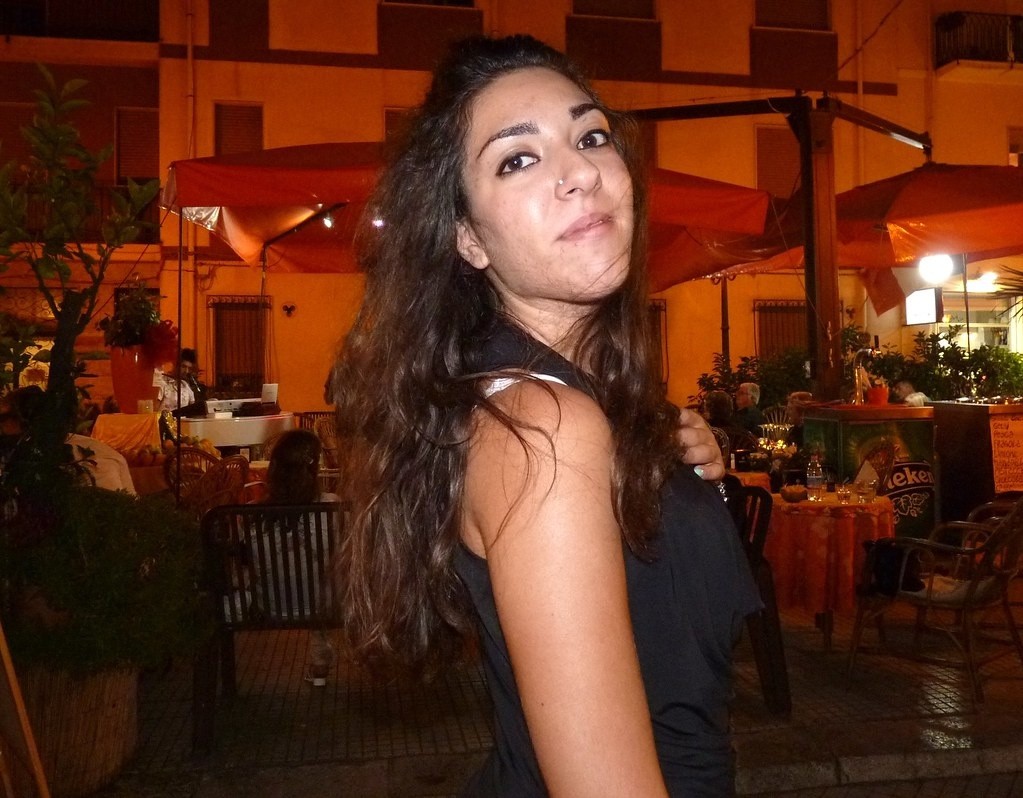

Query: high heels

[304,662,327,688]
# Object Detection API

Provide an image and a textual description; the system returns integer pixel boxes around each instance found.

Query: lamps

[918,252,964,287]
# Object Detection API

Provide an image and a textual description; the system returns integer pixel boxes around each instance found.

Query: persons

[226,431,347,688]
[304,33,765,798]
[784,391,816,487]
[732,383,782,495]
[702,390,760,453]
[893,380,916,403]
[160,348,196,413]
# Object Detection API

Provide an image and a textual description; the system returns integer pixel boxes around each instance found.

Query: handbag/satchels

[865,539,924,593]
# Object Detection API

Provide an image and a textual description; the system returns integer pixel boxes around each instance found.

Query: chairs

[718,474,792,718]
[165,411,339,513]
[843,491,1023,703]
[707,404,791,468]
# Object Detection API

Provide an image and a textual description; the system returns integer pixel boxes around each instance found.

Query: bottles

[807,456,823,502]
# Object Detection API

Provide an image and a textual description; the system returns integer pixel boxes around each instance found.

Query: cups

[856,479,877,505]
[834,483,852,504]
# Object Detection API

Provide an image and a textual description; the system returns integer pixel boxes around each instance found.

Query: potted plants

[864,343,908,406]
[93,277,178,414]
[0,65,216,798]
[924,345,1023,569]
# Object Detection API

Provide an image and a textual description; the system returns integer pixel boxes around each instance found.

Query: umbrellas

[693,162,1023,315]
[164,134,809,302]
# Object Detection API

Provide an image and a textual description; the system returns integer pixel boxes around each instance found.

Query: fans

[280,302,299,318]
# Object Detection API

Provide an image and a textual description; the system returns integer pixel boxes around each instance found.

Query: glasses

[735,390,753,397]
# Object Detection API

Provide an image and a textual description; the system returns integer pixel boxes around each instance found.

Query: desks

[731,472,771,500]
[745,484,895,652]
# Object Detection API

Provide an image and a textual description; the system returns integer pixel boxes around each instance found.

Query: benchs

[193,501,352,754]
